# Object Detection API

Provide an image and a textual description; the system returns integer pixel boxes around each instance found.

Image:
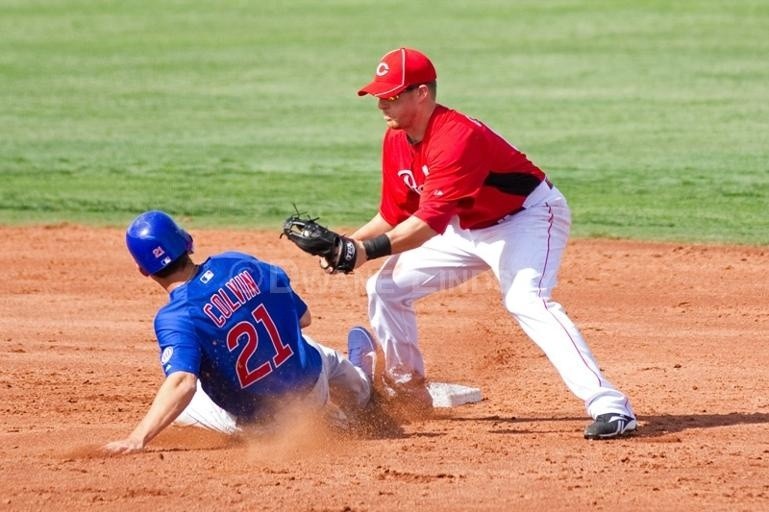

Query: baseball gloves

[284,217,357,276]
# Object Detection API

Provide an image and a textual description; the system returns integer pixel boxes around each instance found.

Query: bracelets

[361,233,393,260]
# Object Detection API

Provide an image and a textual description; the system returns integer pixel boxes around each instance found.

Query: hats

[356,46,438,103]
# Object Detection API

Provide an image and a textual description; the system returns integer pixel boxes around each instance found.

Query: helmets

[125,208,195,278]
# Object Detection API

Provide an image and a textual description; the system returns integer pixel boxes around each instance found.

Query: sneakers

[346,325,387,397]
[581,412,640,443]
[392,381,436,412]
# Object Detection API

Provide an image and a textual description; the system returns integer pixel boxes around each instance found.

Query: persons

[281,46,637,440]
[94,210,380,453]
